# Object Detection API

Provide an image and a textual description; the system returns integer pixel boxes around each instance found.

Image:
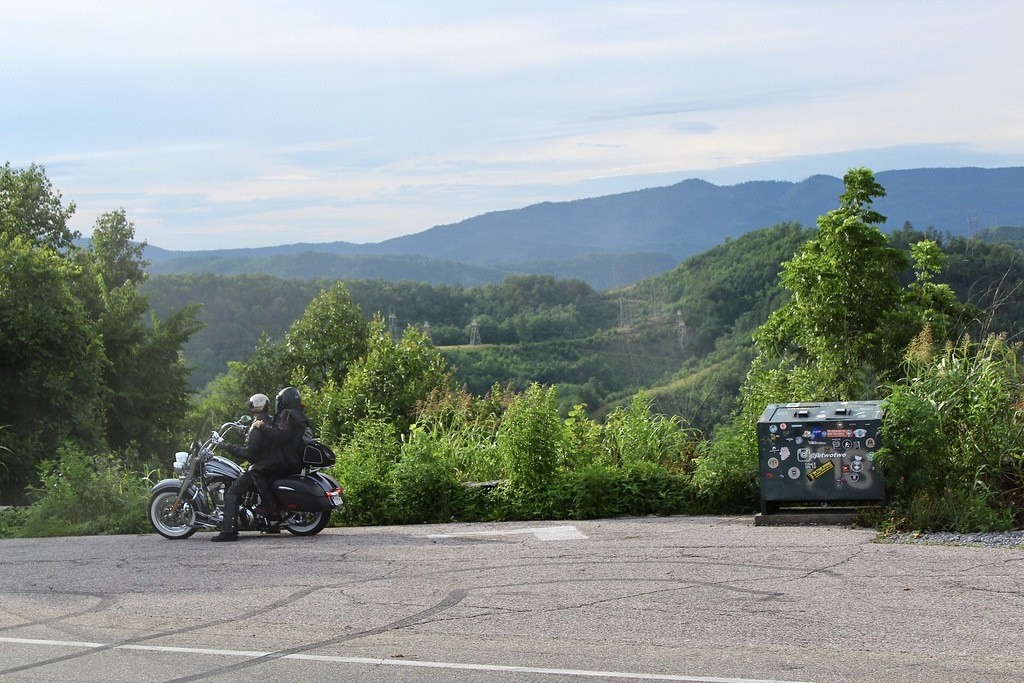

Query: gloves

[211,430,224,444]
[235,421,245,431]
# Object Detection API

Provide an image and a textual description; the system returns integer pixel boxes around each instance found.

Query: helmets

[249,394,270,414]
[275,387,301,412]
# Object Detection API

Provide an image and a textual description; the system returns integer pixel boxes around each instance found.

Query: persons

[211,393,280,541]
[248,386,314,517]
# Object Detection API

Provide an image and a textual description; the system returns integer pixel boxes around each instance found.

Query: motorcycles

[147,415,343,539]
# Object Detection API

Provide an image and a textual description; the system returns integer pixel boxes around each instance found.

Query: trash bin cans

[756,400,889,516]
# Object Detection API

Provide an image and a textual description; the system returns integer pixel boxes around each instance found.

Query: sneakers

[212,532,237,541]
[265,530,279,534]
